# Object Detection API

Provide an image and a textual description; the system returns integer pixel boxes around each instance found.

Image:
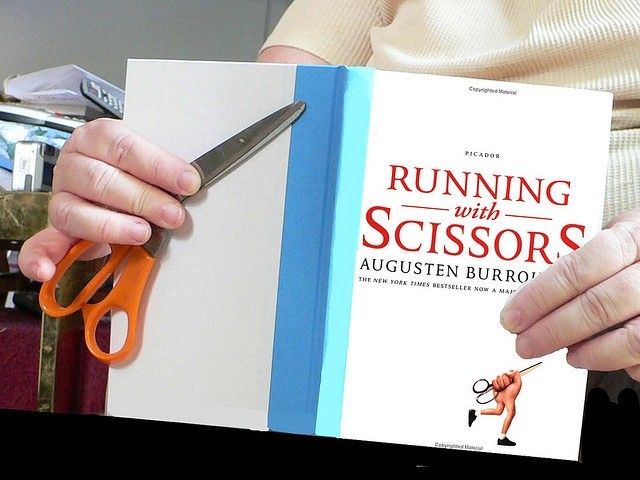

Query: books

[5,64,125,105]
[104,57,614,465]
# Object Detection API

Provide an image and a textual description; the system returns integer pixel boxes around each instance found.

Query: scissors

[473,362,542,404]
[40,101,305,364]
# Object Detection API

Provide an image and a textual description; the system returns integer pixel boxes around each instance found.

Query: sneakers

[469,409,477,427]
[498,437,516,446]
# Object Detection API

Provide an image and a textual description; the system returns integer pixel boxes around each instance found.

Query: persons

[19,1,640,383]
